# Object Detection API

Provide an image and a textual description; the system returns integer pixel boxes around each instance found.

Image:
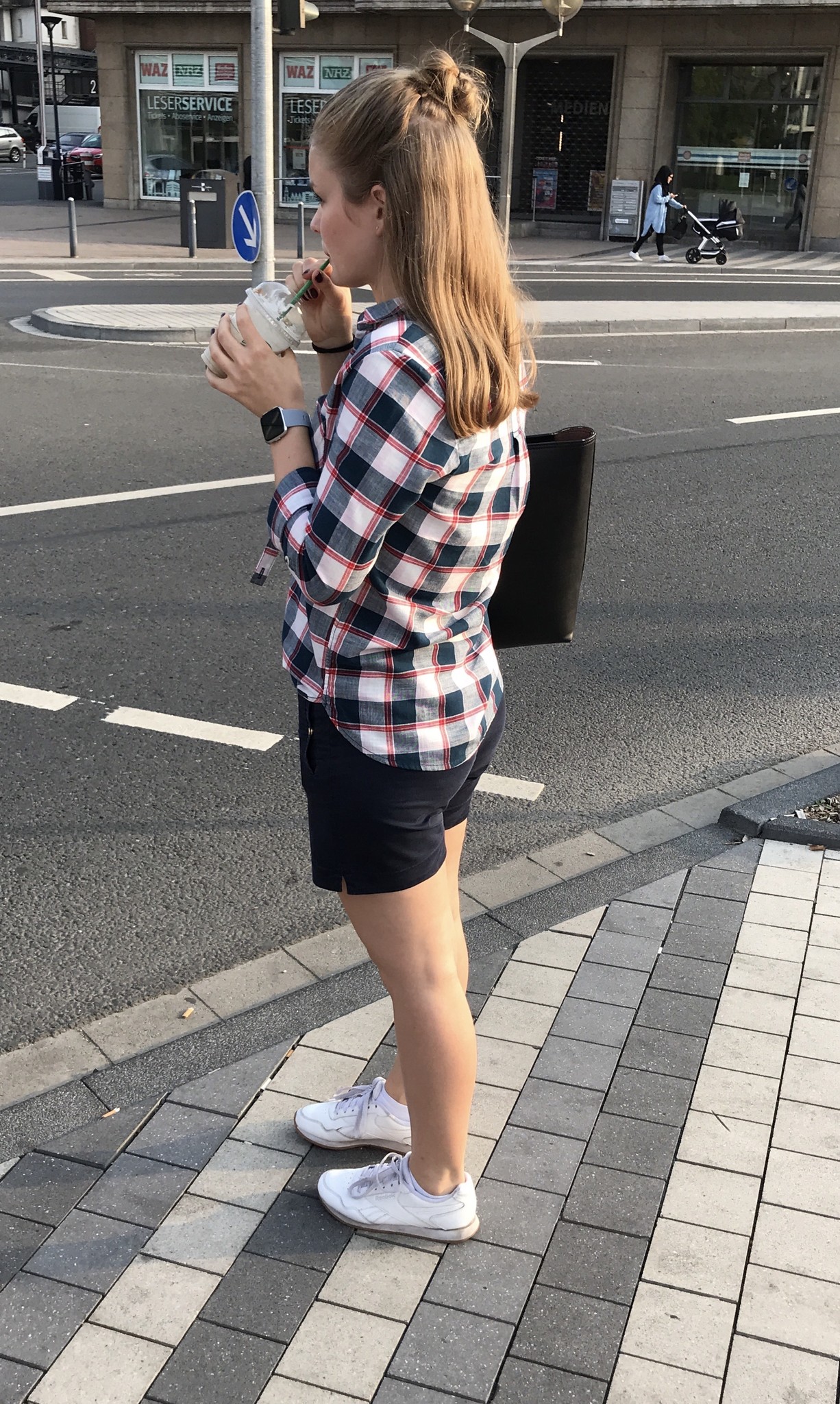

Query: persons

[204,48,545,1244]
[629,165,679,263]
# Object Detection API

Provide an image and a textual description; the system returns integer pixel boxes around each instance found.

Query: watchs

[258,405,313,444]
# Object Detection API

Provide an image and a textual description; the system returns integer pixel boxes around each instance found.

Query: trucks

[22,104,102,146]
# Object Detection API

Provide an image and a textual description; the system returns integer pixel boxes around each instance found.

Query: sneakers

[294,1075,413,1153]
[318,1152,479,1244]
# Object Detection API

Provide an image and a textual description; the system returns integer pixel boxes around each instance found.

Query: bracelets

[311,333,355,354]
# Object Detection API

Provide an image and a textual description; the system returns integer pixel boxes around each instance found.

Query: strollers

[681,198,746,265]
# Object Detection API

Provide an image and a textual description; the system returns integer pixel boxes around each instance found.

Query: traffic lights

[277,0,319,31]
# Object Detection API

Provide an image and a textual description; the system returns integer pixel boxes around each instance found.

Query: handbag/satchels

[671,207,690,240]
[487,426,596,650]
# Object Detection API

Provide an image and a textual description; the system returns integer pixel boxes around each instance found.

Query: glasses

[669,176,673,179]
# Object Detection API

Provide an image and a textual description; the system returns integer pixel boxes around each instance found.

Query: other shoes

[658,255,673,262]
[629,251,642,262]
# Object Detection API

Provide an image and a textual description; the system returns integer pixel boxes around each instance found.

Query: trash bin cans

[180,169,244,250]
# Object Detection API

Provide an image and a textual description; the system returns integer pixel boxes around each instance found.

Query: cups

[200,281,309,379]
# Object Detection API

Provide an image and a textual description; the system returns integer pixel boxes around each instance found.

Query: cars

[143,154,197,181]
[68,132,103,176]
[0,126,26,162]
[49,131,96,168]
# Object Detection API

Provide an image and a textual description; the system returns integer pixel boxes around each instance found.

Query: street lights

[39,13,65,162]
[448,0,585,257]
